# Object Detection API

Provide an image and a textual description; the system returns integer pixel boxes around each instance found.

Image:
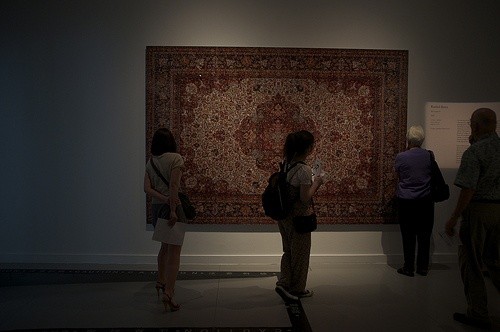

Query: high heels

[162,296,180,311]
[155,282,166,297]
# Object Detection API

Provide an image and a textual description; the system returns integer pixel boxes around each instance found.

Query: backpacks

[262,160,306,221]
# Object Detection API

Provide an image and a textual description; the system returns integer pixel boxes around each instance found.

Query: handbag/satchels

[429,150,450,202]
[178,192,196,220]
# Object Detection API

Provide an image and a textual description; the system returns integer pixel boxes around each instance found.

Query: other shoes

[397,268,415,277]
[275,286,300,304]
[418,269,429,277]
[453,311,486,327]
[297,290,312,297]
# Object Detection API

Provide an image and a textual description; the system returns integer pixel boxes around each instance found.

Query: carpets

[144,45,409,226]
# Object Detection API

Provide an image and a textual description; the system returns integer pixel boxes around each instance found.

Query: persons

[393,125,436,276]
[144,127,186,313]
[275,130,326,303]
[445,107,500,329]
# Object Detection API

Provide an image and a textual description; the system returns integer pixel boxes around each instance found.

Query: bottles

[311,167,314,183]
